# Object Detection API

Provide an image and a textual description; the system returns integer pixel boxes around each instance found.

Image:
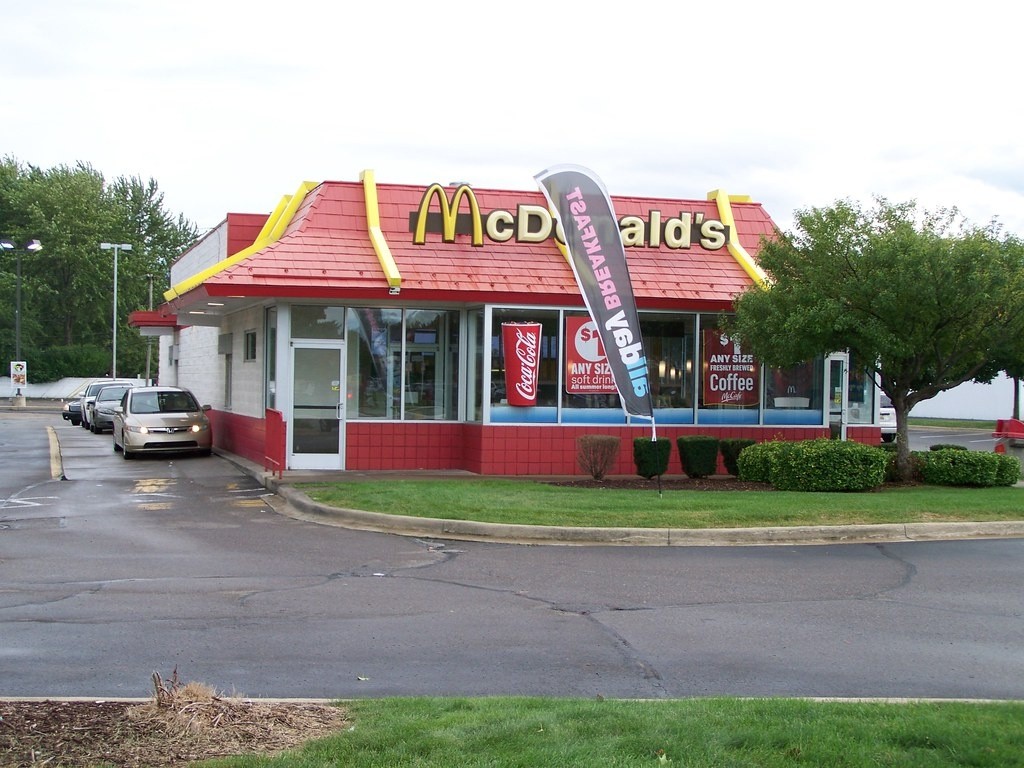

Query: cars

[113,386,213,460]
[62,381,142,434]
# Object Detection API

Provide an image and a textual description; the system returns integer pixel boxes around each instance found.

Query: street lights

[0,239,43,406]
[100,243,132,381]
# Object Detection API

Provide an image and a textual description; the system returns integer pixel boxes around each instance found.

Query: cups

[501,323,543,406]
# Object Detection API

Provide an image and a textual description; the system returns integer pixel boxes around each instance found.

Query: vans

[880,390,897,442]
